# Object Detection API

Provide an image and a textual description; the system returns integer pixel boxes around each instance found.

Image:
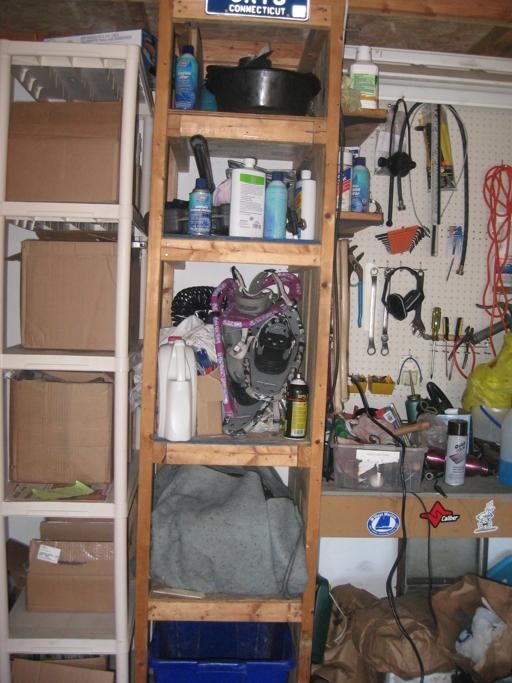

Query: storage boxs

[328,420,430,495]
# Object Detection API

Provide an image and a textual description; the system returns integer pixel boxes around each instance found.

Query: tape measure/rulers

[430,103,440,225]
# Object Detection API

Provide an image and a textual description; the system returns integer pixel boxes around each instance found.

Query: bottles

[405,371,421,423]
[284,373,309,441]
[189,178,212,236]
[228,157,318,240]
[425,446,493,476]
[174,45,199,110]
[348,43,381,108]
[445,418,468,486]
[348,155,371,214]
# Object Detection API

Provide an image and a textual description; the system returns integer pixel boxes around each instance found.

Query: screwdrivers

[431,307,463,380]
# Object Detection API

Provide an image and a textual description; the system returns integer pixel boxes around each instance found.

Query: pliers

[348,246,364,328]
[448,326,474,369]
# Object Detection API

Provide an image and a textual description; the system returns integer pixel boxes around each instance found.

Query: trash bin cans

[148,621,296,683]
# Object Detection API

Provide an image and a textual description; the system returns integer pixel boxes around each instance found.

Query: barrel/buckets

[498,409,512,486]
[156,336,197,442]
[470,405,510,444]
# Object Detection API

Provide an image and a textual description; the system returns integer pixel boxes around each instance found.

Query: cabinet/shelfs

[0,1,343,683]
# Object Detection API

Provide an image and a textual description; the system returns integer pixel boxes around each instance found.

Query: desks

[322,460,512,558]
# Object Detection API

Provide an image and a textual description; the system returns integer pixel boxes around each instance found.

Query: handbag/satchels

[126,433,313,600]
